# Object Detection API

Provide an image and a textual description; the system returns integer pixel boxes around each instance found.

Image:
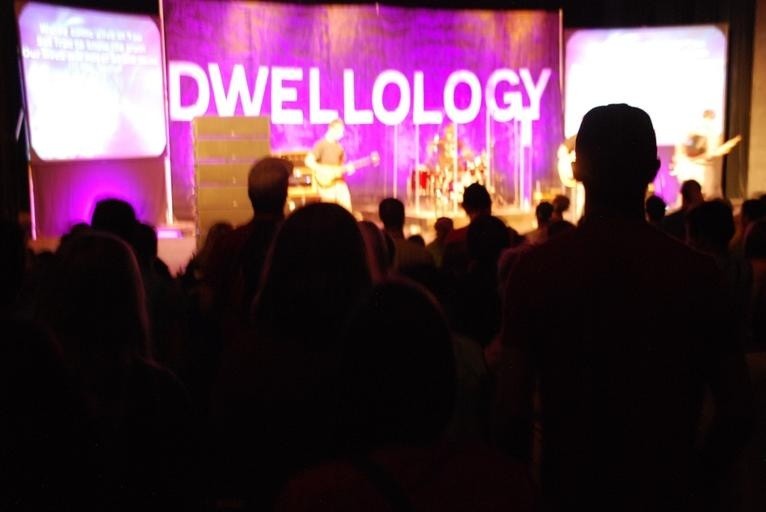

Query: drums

[411,166,428,196]
[467,165,484,177]
[448,179,464,202]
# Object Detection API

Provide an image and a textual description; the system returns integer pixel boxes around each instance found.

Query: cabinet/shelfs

[190,114,271,253]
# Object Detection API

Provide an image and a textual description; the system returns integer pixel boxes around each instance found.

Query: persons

[0,102,766,510]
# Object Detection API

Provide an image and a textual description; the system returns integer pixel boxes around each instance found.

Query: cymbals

[427,140,447,145]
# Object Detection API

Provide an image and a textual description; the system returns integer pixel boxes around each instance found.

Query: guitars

[315,151,380,189]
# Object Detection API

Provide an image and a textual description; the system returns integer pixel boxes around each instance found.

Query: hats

[248,157,295,185]
[575,103,656,155]
